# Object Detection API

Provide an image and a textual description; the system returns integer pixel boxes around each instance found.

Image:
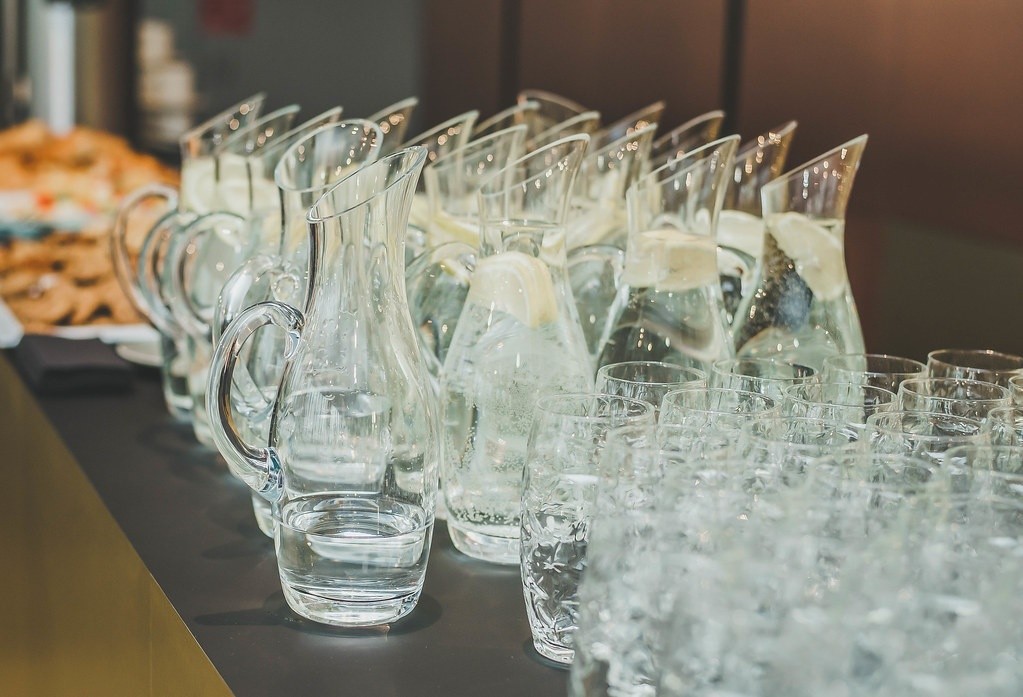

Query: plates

[116,340,165,369]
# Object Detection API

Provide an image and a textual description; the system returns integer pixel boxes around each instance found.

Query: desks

[2,331,581,697]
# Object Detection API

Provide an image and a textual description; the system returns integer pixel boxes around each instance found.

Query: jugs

[110,89,873,627]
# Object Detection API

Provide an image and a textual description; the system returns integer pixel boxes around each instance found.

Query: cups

[518,350,1021,695]
